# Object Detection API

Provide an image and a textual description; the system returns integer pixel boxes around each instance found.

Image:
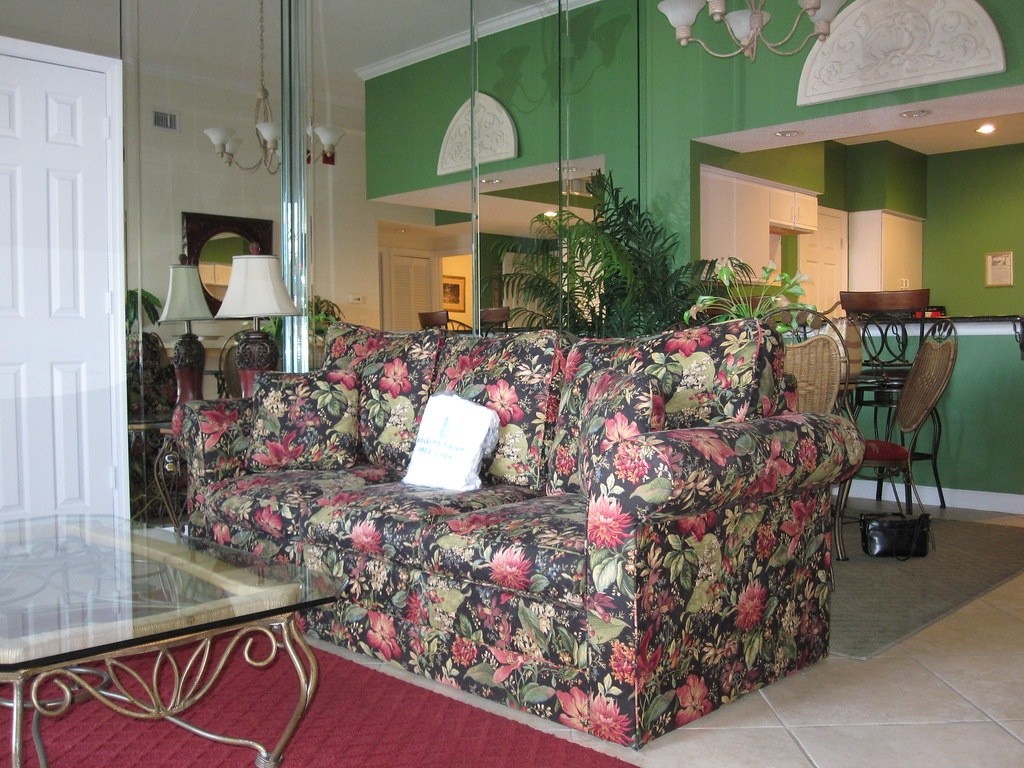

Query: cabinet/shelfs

[699,164,818,284]
[848,211,923,294]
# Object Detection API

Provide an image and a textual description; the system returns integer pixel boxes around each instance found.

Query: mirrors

[121,0,641,527]
[181,212,273,320]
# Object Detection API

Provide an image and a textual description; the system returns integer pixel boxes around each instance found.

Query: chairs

[834,316,959,562]
[760,308,850,416]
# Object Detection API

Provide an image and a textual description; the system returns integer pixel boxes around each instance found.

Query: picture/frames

[443,274,466,312]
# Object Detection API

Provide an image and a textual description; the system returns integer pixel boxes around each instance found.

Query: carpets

[0,609,640,768]
[824,504,1024,662]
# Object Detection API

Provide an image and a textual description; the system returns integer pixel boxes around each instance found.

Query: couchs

[125,330,187,517]
[171,321,865,750]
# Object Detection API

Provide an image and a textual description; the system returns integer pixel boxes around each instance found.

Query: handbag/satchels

[859,511,932,561]
[826,316,863,381]
[403,394,496,492]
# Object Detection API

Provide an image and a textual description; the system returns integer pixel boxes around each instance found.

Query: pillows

[403,394,500,493]
[254,369,361,472]
[577,369,666,499]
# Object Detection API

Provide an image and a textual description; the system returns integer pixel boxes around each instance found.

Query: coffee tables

[0,521,348,768]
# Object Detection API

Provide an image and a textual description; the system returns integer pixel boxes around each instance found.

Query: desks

[128,420,173,527]
[154,426,188,532]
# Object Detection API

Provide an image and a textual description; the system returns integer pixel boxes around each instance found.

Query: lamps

[658,0,848,61]
[158,265,214,408]
[214,254,303,397]
[202,0,345,175]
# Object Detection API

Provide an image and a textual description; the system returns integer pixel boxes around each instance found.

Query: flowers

[683,258,816,338]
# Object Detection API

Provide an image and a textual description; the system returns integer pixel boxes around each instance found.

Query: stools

[840,288,947,511]
[418,307,511,333]
